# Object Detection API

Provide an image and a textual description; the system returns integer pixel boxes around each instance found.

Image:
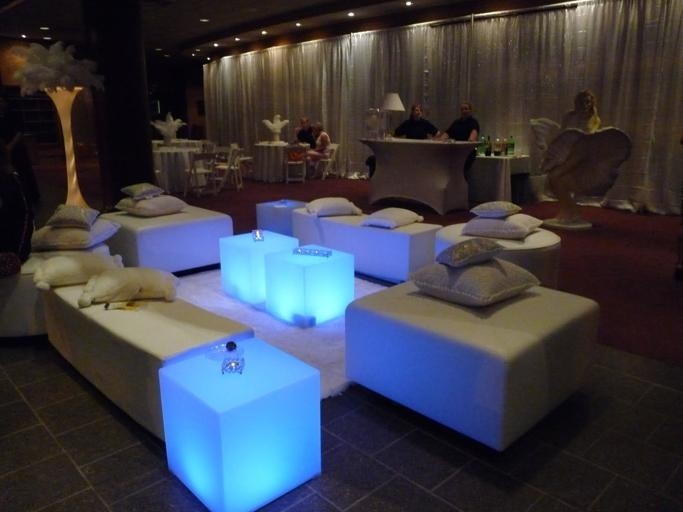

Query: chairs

[7,133,103,258]
[285,145,308,183]
[184,152,218,198]
[212,149,243,191]
[209,142,239,186]
[236,143,252,178]
[312,142,339,180]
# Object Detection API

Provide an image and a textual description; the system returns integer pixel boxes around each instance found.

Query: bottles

[479,135,486,153]
[507,136,515,155]
[502,138,508,156]
[494,137,501,155]
[485,135,492,156]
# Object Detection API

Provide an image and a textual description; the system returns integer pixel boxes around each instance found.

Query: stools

[0,242,111,339]
[434,223,561,290]
[100,203,233,274]
[347,283,600,466]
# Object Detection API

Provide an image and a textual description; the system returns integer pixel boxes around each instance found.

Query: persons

[393,105,441,140]
[560,89,600,134]
[441,102,479,168]
[304,122,330,180]
[296,116,316,148]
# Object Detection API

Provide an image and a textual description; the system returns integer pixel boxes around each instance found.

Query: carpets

[172,268,388,399]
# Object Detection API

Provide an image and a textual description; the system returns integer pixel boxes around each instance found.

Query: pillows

[434,237,505,269]
[31,218,122,252]
[460,213,544,239]
[78,266,181,308]
[114,194,190,216]
[120,182,166,202]
[470,200,522,218]
[409,256,541,308]
[32,255,125,291]
[47,204,100,231]
[305,196,362,220]
[361,207,424,229]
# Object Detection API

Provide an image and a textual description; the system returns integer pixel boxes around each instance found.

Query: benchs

[293,207,443,284]
[40,268,254,444]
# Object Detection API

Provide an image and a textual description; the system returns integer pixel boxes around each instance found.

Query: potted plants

[5,43,105,208]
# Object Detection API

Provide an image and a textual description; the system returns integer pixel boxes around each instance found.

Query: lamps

[381,92,406,138]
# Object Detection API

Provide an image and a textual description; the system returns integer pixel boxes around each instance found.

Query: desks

[154,148,205,194]
[471,153,532,202]
[356,138,484,215]
[152,138,214,165]
[253,142,311,184]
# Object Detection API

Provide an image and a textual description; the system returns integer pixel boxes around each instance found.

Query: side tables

[218,229,300,311]
[159,338,322,512]
[265,245,355,327]
[256,199,306,236]
